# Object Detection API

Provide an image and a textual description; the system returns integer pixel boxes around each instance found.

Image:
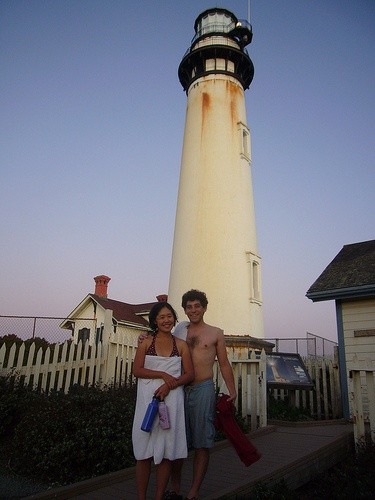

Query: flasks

[158,398,170,430]
[141,394,160,433]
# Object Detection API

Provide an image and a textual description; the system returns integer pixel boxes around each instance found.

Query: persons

[138,288,236,500]
[131,302,195,500]
